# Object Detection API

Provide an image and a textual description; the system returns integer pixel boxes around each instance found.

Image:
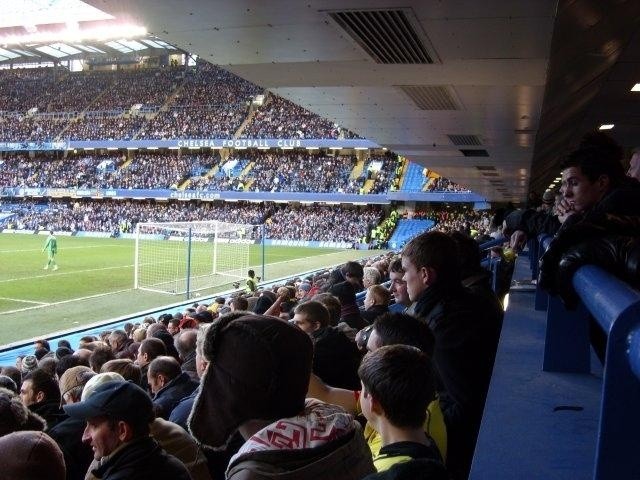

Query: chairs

[399,163,424,192]
[387,219,435,249]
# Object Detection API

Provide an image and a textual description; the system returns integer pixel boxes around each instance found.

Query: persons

[0,56,639,480]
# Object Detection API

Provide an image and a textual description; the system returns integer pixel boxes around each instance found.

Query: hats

[57,381,154,421]
[0,431,66,480]
[327,281,356,305]
[188,311,315,450]
[58,365,97,407]
[253,295,274,314]
[0,387,47,430]
[298,283,312,291]
[21,354,39,371]
[80,373,126,403]
